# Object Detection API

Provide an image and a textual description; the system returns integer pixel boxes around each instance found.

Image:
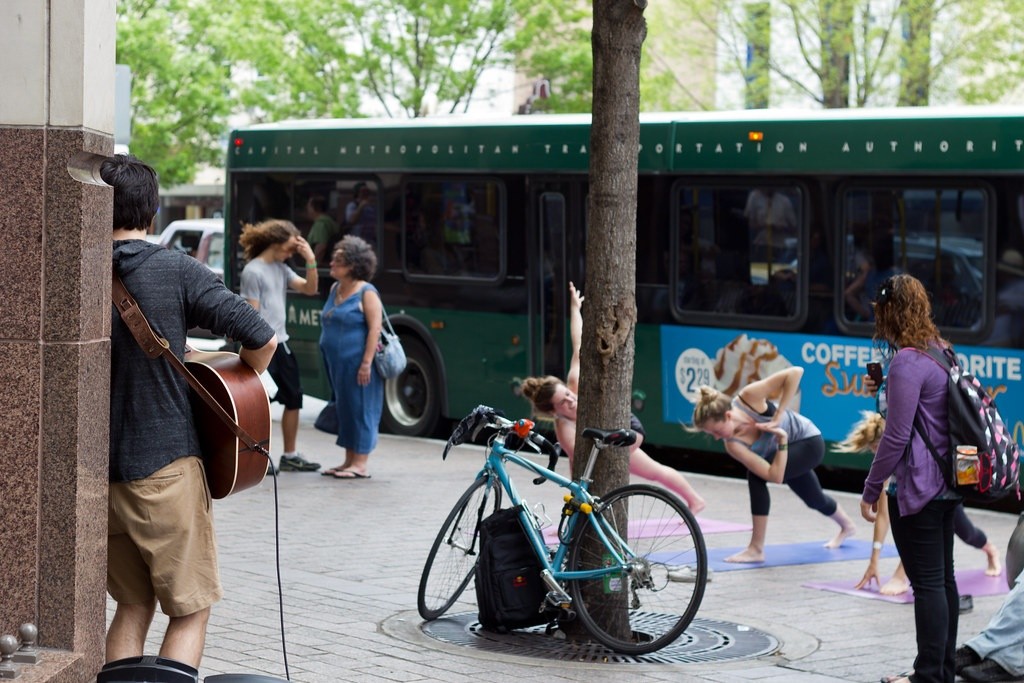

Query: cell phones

[867,363,883,392]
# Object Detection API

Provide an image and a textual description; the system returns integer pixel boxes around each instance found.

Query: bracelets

[779,444,788,450]
[305,262,317,268]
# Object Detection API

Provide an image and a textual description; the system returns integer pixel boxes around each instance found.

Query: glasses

[869,425,882,445]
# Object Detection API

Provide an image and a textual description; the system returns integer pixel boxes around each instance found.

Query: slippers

[321,466,372,480]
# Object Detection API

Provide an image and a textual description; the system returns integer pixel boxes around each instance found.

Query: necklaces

[338,283,347,303]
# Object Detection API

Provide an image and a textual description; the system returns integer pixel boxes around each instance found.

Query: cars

[158,219,224,279]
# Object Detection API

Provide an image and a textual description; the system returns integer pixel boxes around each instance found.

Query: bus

[222,106,1024,505]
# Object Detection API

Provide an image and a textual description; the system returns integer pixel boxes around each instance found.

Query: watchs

[871,541,883,550]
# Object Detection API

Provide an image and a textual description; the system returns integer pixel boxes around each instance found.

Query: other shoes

[880,671,910,683]
[669,566,713,582]
[958,595,973,614]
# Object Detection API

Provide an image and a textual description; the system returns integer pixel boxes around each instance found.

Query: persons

[97,156,278,670]
[714,202,796,318]
[845,251,984,329]
[522,282,705,524]
[239,220,320,472]
[443,176,475,278]
[953,569,1024,683]
[181,236,199,257]
[314,235,384,478]
[829,273,1001,683]
[995,250,1024,339]
[745,179,795,228]
[833,413,1001,595]
[408,214,500,279]
[306,183,383,268]
[695,366,855,563]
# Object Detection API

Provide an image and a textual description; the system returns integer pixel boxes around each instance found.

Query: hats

[997,249,1024,277]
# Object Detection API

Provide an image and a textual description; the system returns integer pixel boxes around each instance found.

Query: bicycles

[418,405,708,654]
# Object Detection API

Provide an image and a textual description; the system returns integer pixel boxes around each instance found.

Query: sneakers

[278,456,321,472]
[954,646,1024,683]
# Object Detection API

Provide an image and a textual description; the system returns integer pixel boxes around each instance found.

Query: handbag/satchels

[314,400,339,435]
[375,305,408,379]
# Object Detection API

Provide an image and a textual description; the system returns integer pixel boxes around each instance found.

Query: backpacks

[474,506,562,635]
[442,200,470,243]
[885,343,1022,501]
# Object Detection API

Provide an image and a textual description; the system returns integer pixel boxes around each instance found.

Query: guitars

[181,342,272,499]
